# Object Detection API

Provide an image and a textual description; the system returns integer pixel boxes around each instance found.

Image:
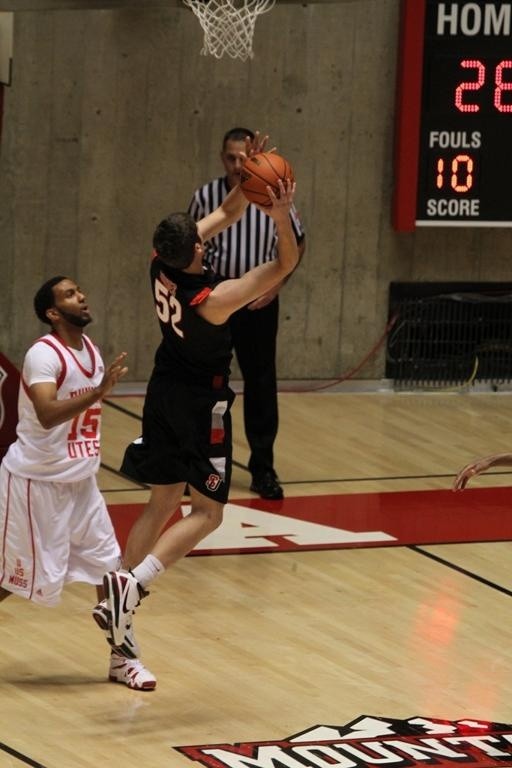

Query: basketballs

[239,151,294,206]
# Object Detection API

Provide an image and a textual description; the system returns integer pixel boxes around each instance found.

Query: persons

[187,129,307,499]
[93,131,299,660]
[1,276,158,692]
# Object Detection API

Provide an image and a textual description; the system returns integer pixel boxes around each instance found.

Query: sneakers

[91,570,158,691]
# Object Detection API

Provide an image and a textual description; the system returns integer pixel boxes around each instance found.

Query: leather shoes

[249,468,283,500]
